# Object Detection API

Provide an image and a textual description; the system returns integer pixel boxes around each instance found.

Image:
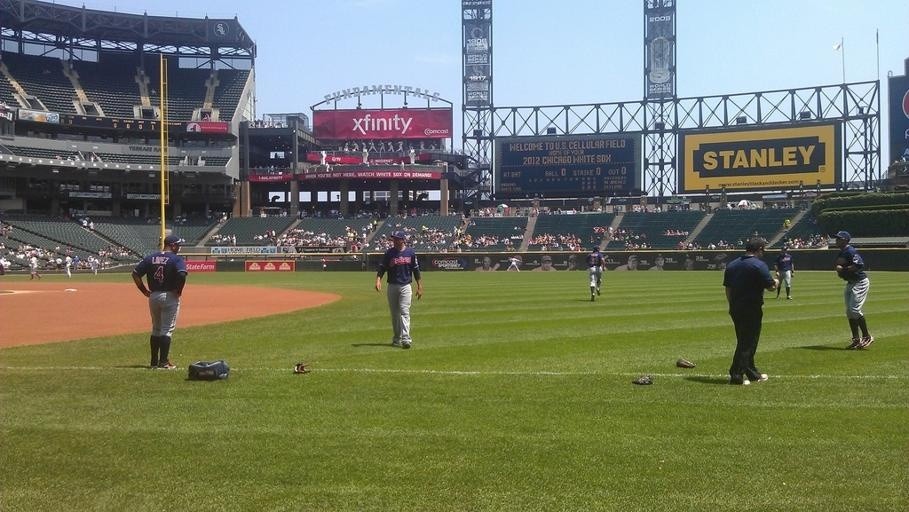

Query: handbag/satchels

[188,360,230,380]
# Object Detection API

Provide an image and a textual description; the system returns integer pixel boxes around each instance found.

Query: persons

[131,234,188,369]
[98,245,129,258]
[648,256,666,271]
[376,224,526,252]
[831,230,875,349]
[776,245,795,300]
[1,241,33,268]
[352,140,360,151]
[211,232,238,245]
[29,254,41,279]
[781,232,832,250]
[531,256,558,272]
[388,142,395,152]
[375,230,425,350]
[614,255,639,271]
[36,244,62,270]
[474,256,495,272]
[378,140,386,153]
[0,220,15,239]
[396,141,404,152]
[254,230,271,242]
[271,225,370,248]
[217,211,231,225]
[87,255,101,276]
[368,140,377,152]
[586,245,605,301]
[724,237,781,385]
[529,233,582,254]
[592,223,653,251]
[65,255,77,278]
[664,229,691,237]
[674,241,743,251]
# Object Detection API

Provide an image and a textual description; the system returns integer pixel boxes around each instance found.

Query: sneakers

[757,372,769,383]
[403,341,411,349]
[741,379,750,385]
[856,336,874,350]
[149,361,158,369]
[392,340,401,346]
[158,361,176,371]
[848,338,862,350]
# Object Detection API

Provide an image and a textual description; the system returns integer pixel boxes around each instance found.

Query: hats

[593,244,600,249]
[831,230,852,241]
[746,238,766,252]
[781,245,789,250]
[391,231,406,240]
[164,235,186,246]
